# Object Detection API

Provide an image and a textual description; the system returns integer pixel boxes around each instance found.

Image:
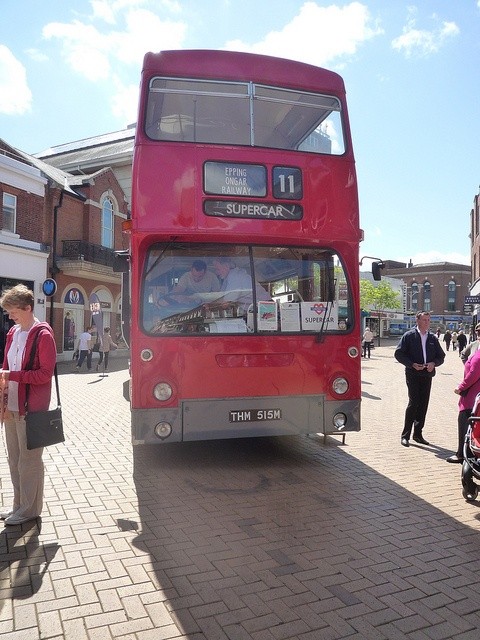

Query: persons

[446,345,480,463]
[460,321,480,365]
[1,283,57,526]
[456,330,467,357]
[171,259,221,305]
[96,327,119,372]
[450,332,458,351]
[435,326,441,339]
[394,311,445,447]
[363,327,373,359]
[443,330,452,351]
[202,255,275,318]
[76,327,93,372]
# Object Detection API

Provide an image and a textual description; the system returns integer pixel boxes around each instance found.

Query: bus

[111,50,386,449]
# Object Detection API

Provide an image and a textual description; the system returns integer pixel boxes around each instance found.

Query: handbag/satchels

[24,328,65,450]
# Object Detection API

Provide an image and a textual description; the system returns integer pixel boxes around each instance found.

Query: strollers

[461,390,480,501]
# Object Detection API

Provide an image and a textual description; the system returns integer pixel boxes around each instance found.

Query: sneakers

[0,509,16,518]
[5,513,38,525]
[446,455,464,462]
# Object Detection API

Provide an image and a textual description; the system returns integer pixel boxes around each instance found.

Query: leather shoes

[413,437,429,445]
[401,437,409,446]
